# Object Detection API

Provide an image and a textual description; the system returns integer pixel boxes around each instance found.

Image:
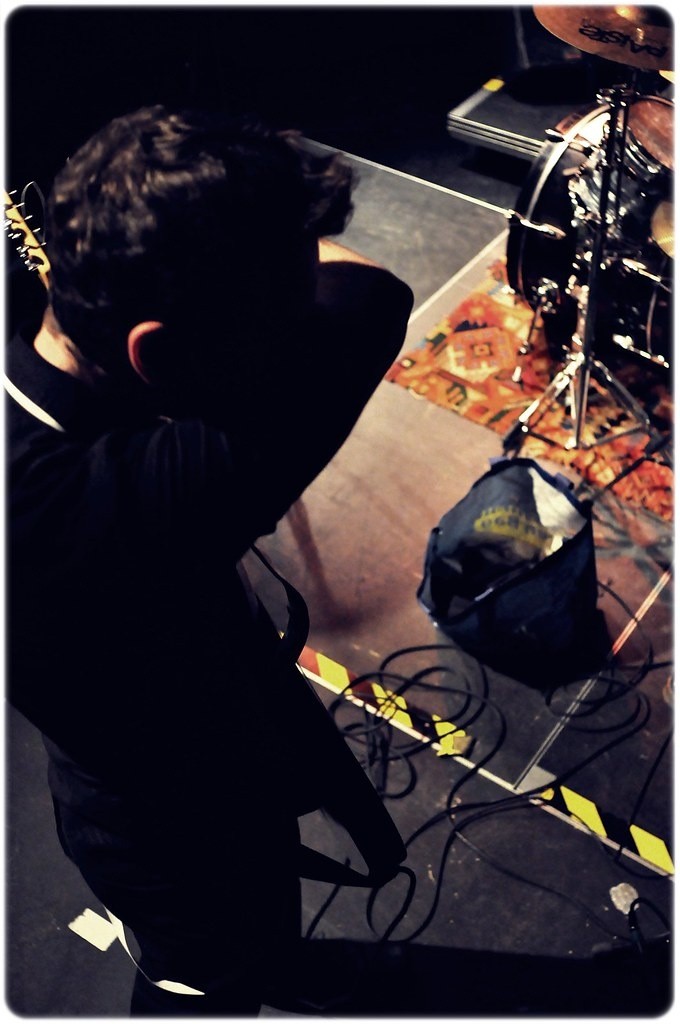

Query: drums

[505,84,674,374]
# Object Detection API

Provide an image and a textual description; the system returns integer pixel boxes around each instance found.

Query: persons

[7,104,415,1018]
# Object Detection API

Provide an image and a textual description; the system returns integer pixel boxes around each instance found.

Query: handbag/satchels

[416,457,598,682]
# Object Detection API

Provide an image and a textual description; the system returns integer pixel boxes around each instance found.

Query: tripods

[500,64,674,475]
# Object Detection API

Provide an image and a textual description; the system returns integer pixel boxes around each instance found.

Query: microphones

[628,911,664,993]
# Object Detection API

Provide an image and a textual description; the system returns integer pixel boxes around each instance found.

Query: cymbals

[533,5,675,72]
